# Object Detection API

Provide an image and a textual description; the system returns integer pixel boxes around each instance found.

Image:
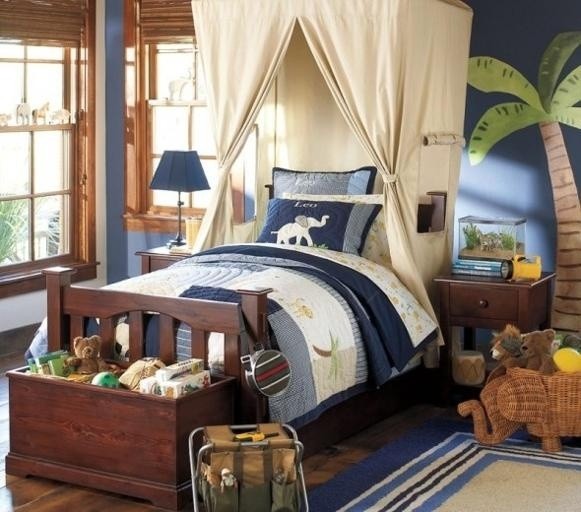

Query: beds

[24,216,452,438]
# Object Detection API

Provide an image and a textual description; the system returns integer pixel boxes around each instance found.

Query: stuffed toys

[510,329,557,376]
[67,336,107,375]
[489,324,527,368]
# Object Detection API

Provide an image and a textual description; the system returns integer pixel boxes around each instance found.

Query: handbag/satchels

[240,349,291,398]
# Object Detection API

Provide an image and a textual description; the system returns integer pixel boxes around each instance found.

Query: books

[28,350,75,378]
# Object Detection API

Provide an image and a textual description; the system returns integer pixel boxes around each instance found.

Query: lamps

[149,149,211,249]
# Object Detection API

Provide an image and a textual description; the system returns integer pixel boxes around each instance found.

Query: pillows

[254,199,384,257]
[270,166,378,196]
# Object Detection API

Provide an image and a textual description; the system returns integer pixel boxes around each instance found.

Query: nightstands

[433,271,557,370]
[134,247,189,276]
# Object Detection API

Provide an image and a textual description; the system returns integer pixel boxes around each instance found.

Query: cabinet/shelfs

[188,423,311,512]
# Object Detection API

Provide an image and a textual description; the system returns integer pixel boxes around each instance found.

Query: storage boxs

[5,363,236,512]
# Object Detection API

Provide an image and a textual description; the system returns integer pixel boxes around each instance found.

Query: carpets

[301,416,581,511]
